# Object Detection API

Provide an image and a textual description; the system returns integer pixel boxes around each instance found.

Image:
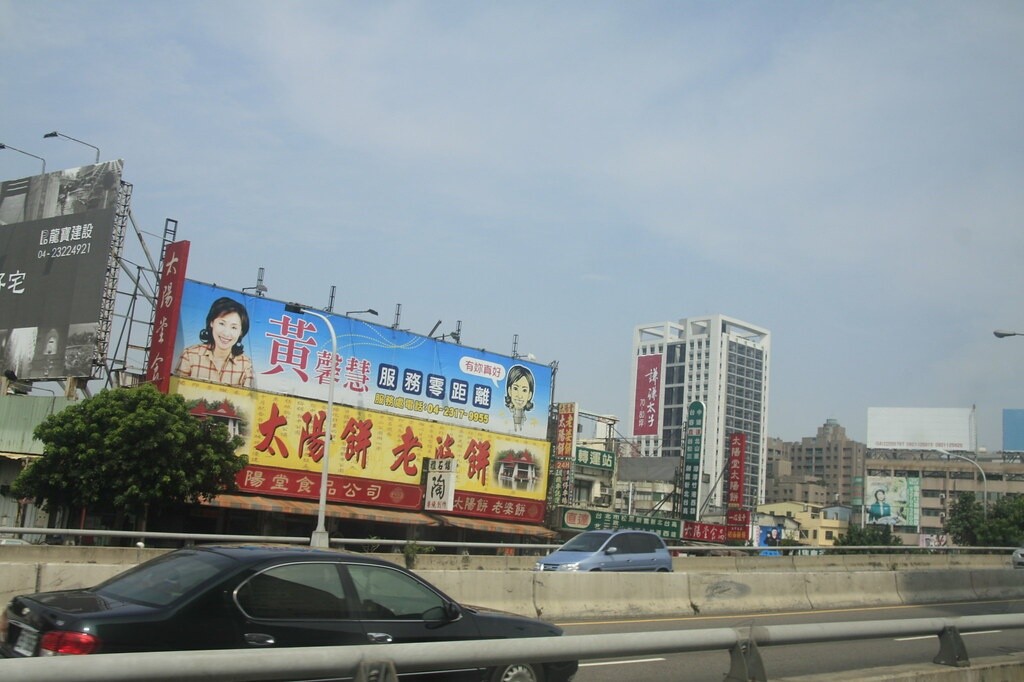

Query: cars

[534,527,674,572]
[1011,546,1024,570]
[1,544,578,682]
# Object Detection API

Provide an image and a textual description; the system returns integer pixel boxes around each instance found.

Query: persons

[763,527,781,546]
[175,297,256,389]
[869,489,890,522]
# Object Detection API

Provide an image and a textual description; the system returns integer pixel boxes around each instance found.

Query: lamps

[510,353,535,360]
[434,332,460,340]
[4,369,55,397]
[346,309,378,317]
[0,143,46,174]
[241,285,268,293]
[43,131,100,164]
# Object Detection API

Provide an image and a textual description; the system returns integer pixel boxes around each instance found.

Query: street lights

[283,302,338,550]
[935,448,987,524]
